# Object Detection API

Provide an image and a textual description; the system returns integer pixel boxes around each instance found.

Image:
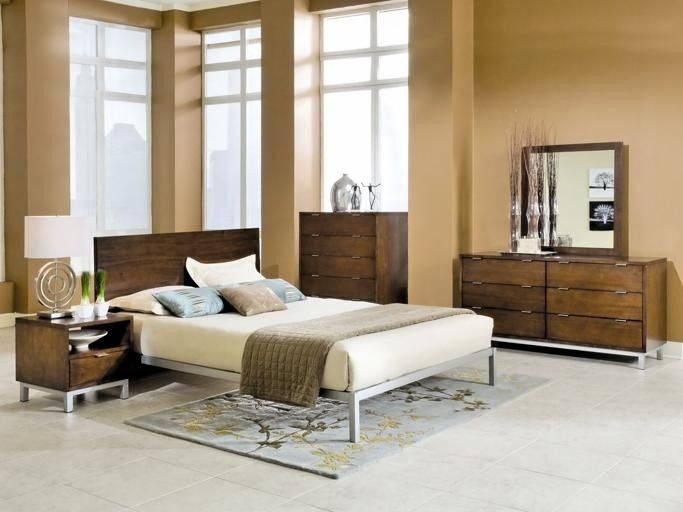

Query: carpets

[126,366,552,477]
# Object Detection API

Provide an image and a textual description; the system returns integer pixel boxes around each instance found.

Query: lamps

[24,215,88,319]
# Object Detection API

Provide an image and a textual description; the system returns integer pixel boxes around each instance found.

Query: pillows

[106,253,307,319]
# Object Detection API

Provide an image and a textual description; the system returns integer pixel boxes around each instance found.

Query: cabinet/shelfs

[299,212,408,303]
[460,249,668,370]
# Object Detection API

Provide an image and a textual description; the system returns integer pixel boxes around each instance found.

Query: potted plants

[95,269,108,320]
[78,270,94,321]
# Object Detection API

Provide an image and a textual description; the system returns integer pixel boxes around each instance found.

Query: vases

[332,174,360,212]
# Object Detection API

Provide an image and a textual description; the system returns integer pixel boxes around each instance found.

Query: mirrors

[521,142,630,260]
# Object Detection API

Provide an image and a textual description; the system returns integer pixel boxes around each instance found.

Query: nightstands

[15,313,133,413]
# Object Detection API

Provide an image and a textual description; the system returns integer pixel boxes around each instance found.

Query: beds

[92,229,495,442]
